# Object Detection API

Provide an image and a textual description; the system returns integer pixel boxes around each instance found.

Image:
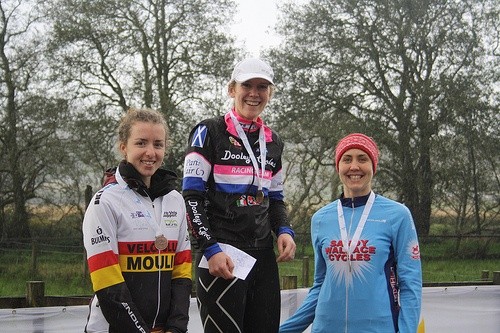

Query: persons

[82,107,193,333]
[280,132,422,333]
[183,57,297,333]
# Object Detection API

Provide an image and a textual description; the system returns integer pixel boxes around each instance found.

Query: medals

[155,236,168,251]
[348,261,352,273]
[256,191,264,204]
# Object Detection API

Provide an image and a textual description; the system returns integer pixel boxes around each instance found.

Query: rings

[291,253,295,258]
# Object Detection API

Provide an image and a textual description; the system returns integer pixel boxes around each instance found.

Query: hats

[336,133,378,176]
[229,57,274,86]
